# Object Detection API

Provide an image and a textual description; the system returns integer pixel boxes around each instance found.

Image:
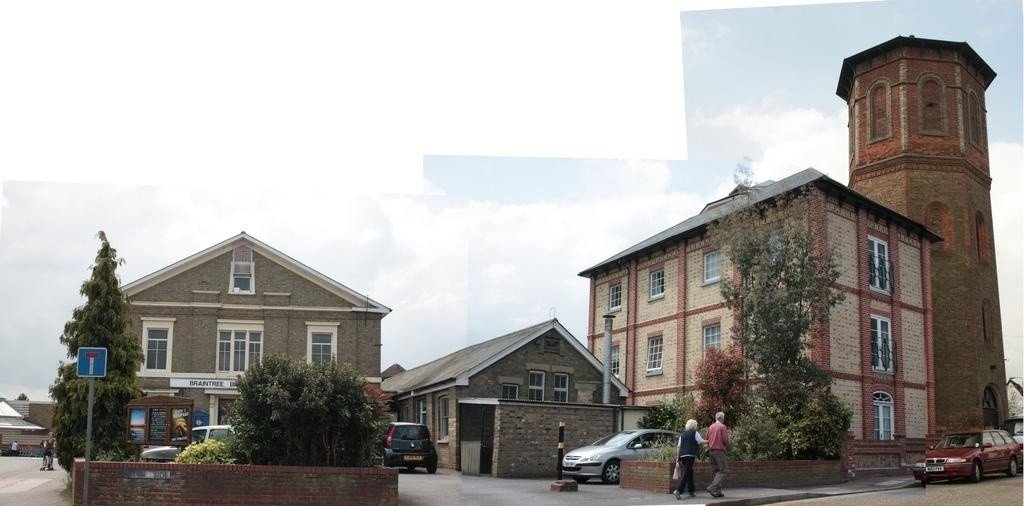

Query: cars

[913,429,1022,483]
[561,427,681,484]
[382,421,437,472]
[1000,415,1023,473]
[139,424,236,463]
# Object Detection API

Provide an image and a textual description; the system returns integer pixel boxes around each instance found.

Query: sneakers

[689,491,696,496]
[673,489,682,501]
[706,488,724,498]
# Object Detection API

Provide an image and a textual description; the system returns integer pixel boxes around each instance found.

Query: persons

[706,410,730,497]
[11,440,18,456]
[43,431,56,470]
[673,419,709,500]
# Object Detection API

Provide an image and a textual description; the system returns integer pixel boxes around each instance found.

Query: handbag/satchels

[672,461,684,480]
